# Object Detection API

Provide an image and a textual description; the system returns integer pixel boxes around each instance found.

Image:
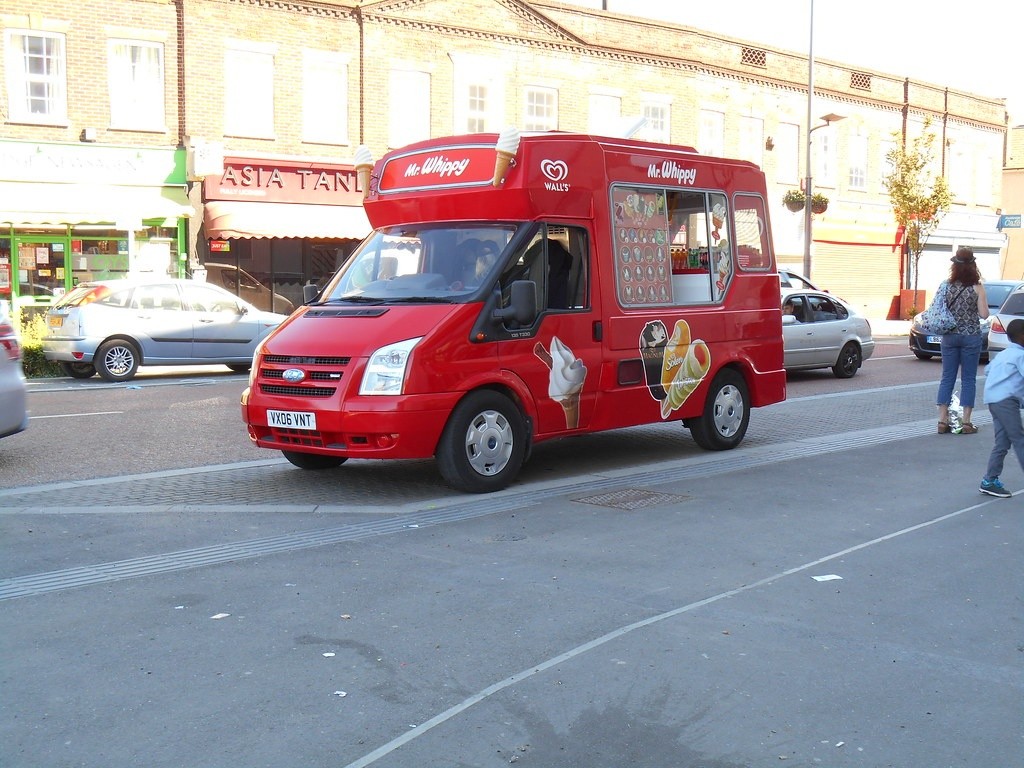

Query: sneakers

[979,478,1012,498]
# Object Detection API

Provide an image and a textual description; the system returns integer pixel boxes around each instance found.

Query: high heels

[938,419,950,433]
[962,422,979,433]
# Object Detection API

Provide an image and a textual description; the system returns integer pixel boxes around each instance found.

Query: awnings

[0,181,196,227]
[203,199,427,259]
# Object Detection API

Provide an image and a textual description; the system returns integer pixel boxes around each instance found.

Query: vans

[238,127,790,492]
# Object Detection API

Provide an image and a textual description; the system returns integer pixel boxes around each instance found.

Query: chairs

[443,237,572,309]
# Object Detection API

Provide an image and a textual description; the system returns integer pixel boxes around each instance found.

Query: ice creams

[493,125,521,186]
[534,334,587,429]
[354,145,375,198]
[713,204,730,294]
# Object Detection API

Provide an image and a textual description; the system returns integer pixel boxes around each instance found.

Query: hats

[950,247,976,264]
[476,240,498,256]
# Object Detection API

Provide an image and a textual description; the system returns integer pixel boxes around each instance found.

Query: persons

[937,247,990,434]
[978,318,1024,498]
[782,299,801,323]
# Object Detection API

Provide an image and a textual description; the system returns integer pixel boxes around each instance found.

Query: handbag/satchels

[921,280,957,335]
[947,391,963,435]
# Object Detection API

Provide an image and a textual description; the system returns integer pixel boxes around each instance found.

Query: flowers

[782,190,806,207]
[812,193,830,204]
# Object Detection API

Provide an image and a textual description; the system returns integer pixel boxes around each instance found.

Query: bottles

[672,247,710,270]
[738,244,762,268]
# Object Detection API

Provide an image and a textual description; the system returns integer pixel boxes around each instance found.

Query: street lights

[802,112,850,291]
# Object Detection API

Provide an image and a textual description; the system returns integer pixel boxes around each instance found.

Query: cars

[774,267,876,378]
[0,306,30,444]
[41,276,289,382]
[987,283,1024,364]
[908,280,1024,362]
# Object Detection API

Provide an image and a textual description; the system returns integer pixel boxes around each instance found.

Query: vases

[812,203,828,214]
[785,202,804,213]
